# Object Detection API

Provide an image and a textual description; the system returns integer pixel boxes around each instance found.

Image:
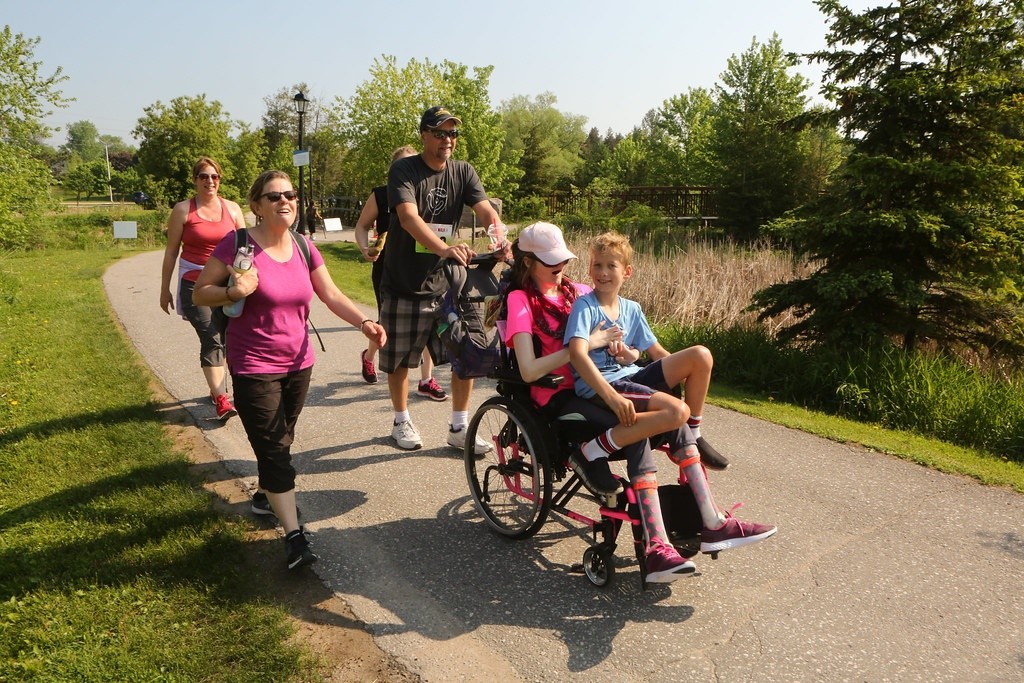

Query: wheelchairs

[442,252,723,593]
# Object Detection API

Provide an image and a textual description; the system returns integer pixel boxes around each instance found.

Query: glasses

[423,129,458,139]
[195,173,220,180]
[529,256,569,268]
[253,191,297,201]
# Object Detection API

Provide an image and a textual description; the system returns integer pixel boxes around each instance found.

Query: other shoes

[310,237,315,241]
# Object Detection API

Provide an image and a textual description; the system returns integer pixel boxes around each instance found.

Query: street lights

[308,143,312,202]
[292,90,311,236]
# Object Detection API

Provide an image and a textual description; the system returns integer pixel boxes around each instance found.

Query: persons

[160,157,246,420]
[562,231,731,496]
[290,198,300,232]
[192,170,387,568]
[354,144,450,401]
[379,107,512,455]
[483,221,778,593]
[305,199,323,241]
[326,194,336,208]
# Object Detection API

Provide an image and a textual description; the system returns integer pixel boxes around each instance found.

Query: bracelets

[226,286,240,302]
[361,319,373,332]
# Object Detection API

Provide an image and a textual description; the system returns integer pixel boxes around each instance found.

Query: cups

[488,222,508,250]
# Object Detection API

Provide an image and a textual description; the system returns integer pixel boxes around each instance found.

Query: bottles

[223,244,255,317]
[437,324,459,351]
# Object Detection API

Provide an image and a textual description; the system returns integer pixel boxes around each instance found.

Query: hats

[518,221,579,265]
[420,106,462,128]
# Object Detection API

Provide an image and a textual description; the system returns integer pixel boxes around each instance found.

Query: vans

[134,190,148,206]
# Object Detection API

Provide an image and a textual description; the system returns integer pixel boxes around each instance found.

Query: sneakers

[252,491,301,520]
[417,377,448,401]
[360,349,379,384]
[568,443,624,495]
[701,502,776,554]
[286,526,317,569]
[212,394,237,420]
[392,418,423,449]
[447,420,494,454]
[646,545,696,582]
[697,436,730,471]
